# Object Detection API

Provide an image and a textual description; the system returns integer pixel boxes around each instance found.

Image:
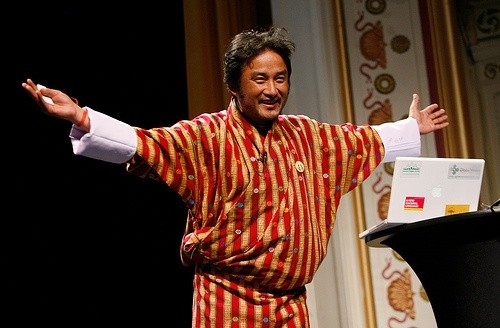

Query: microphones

[262,152,267,166]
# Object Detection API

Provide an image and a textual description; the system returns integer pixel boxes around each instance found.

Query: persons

[23,26,450,328]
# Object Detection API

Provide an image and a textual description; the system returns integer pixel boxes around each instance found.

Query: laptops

[357,156,484,238]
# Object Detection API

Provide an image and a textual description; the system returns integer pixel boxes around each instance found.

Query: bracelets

[76,110,88,127]
